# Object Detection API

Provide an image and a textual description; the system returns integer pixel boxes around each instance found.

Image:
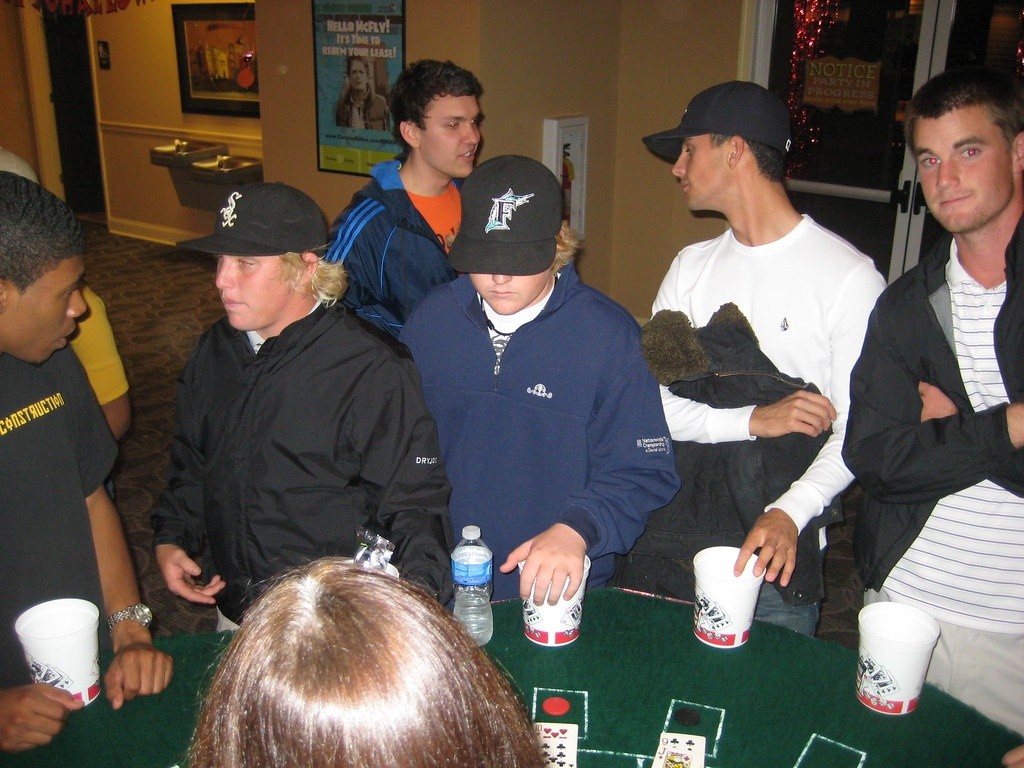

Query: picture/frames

[542,115,589,243]
[171,2,261,119]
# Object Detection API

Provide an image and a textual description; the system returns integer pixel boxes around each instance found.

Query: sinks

[191,154,263,184]
[149,142,227,168]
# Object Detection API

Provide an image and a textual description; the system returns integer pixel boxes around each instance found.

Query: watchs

[108,602,152,634]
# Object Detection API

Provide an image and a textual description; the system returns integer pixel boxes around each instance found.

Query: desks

[0,588,1024,768]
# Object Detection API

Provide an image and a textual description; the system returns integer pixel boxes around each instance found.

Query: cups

[693,547,766,648]
[15,598,100,710]
[856,602,940,716]
[518,555,590,646]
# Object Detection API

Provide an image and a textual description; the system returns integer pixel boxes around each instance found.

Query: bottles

[451,526,492,646]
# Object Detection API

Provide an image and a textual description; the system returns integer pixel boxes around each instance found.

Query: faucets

[217,155,233,169]
[175,139,191,152]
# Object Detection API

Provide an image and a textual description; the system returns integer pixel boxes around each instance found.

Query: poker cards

[530,720,580,768]
[647,731,708,768]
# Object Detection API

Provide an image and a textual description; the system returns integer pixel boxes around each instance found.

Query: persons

[400,154,679,606]
[0,171,174,746]
[607,80,890,636]
[187,563,547,768]
[153,181,456,631]
[842,72,1023,742]
[326,60,483,344]
[0,152,132,439]
[335,57,388,130]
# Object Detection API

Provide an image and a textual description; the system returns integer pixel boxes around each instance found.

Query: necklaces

[480,272,559,337]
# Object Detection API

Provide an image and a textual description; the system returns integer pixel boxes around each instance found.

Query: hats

[176,180,328,257]
[448,155,564,276]
[643,80,792,165]
